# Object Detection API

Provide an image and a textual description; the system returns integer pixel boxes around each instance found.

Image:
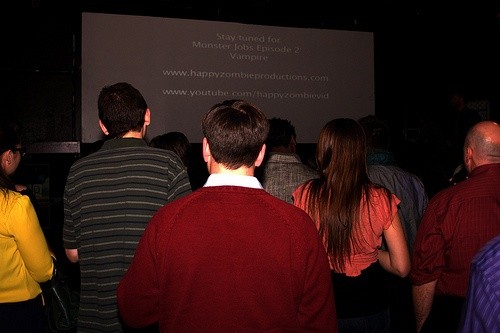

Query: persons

[411,120,500,333]
[290,118,411,333]
[61,80,193,333]
[0,116,61,333]
[357,113,430,249]
[116,98,339,333]
[254,117,324,203]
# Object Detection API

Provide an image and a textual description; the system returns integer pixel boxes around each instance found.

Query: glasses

[10,147,26,156]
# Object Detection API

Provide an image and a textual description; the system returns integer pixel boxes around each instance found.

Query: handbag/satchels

[39,256,75,307]
